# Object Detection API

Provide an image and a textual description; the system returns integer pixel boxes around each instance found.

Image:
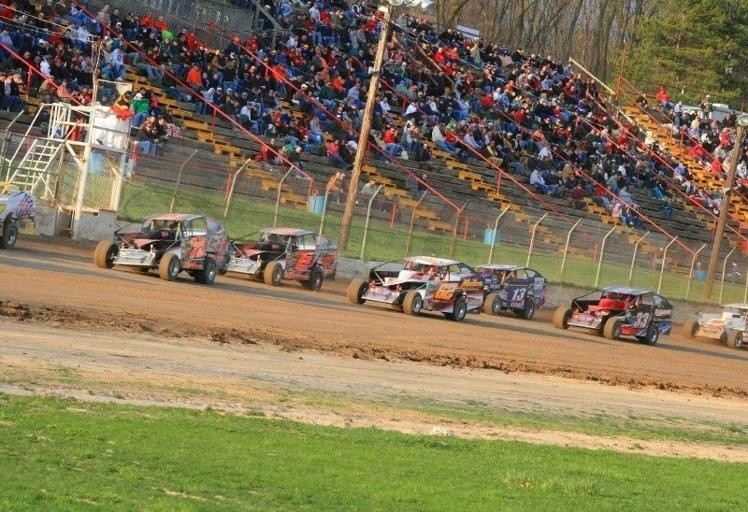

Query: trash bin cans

[484,223,501,245]
[694,271,706,282]
[88,147,103,175]
[309,192,325,215]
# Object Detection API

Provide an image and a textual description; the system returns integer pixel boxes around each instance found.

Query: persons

[254,1,695,226]
[427,266,436,278]
[672,85,748,192]
[1,1,260,164]
[268,234,281,244]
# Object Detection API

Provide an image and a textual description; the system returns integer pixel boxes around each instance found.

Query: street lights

[702,113,748,300]
[339,0,436,252]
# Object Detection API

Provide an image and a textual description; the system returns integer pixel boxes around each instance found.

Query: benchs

[119,62,748,287]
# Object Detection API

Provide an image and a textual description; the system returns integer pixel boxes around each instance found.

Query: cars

[0,182,36,251]
[94,213,338,290]
[348,256,548,324]
[552,285,673,344]
[684,303,747,349]
